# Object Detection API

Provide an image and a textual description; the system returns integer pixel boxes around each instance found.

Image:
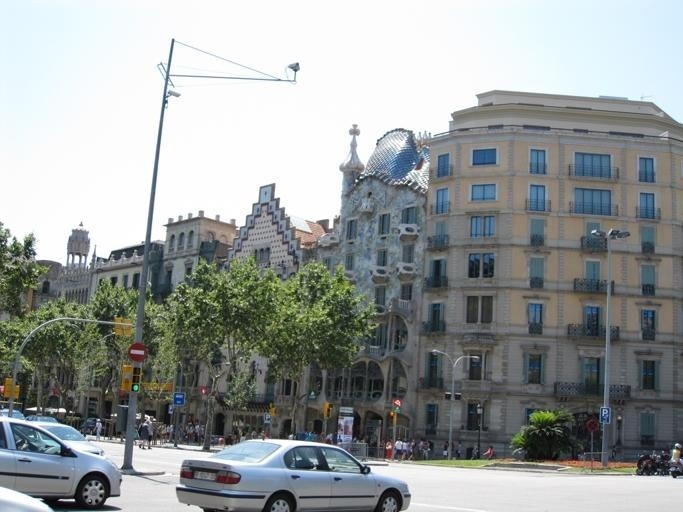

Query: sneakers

[139,443,152,449]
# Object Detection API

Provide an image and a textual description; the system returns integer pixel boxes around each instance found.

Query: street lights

[590,228,631,466]
[432,350,480,462]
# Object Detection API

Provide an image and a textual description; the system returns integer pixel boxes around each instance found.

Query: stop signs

[127,343,148,362]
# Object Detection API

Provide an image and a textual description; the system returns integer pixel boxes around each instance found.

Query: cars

[176,437,412,512]
[0,409,121,509]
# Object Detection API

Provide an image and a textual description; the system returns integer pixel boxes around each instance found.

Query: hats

[97,419,101,421]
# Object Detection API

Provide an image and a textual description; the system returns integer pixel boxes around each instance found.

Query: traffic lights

[121,365,132,393]
[114,315,122,335]
[132,368,141,393]
[324,402,334,418]
[124,319,131,336]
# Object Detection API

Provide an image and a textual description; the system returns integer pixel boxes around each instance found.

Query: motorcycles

[636,450,682,478]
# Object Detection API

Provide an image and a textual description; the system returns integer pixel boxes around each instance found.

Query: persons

[288,430,337,445]
[234,426,241,444]
[483,445,495,458]
[226,434,234,445]
[456,441,462,457]
[470,442,478,460]
[667,443,683,466]
[93,418,102,436]
[442,440,449,459]
[385,437,434,460]
[138,415,205,449]
[245,430,270,440]
